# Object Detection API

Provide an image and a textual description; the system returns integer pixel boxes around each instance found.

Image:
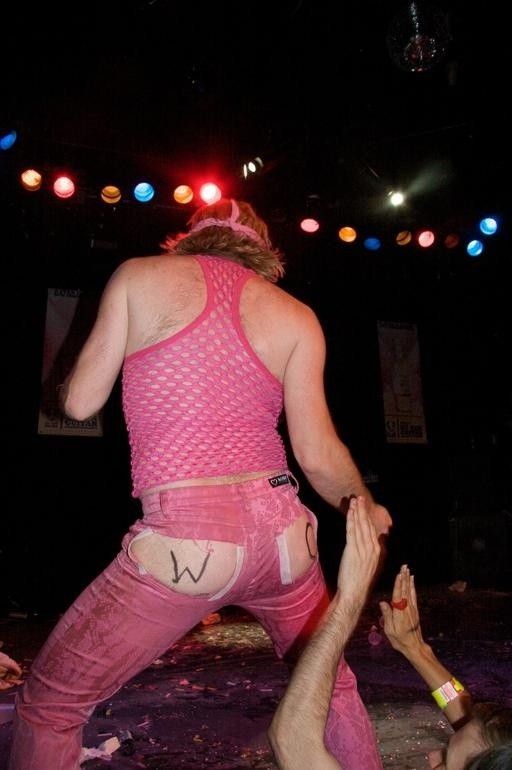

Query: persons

[268,490,512,768]
[0,197,398,769]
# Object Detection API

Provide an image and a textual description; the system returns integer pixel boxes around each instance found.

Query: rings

[390,599,408,610]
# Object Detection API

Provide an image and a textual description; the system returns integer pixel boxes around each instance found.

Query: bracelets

[430,676,467,711]
